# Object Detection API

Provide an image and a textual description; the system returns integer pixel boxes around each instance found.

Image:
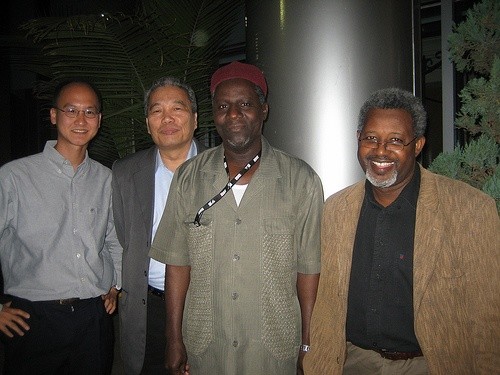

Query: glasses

[55,105,100,119]
[357,131,419,152]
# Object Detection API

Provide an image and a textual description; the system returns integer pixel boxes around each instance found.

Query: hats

[210,61,268,97]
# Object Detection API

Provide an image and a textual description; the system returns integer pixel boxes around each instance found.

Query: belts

[346,339,423,361]
[50,297,80,305]
[148,284,165,297]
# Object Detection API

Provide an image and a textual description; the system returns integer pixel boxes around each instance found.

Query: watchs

[113,284,121,290]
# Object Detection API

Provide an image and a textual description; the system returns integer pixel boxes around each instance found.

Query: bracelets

[301,344,311,353]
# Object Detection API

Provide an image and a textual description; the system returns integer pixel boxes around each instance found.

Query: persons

[111,79,208,375]
[302,88,500,375]
[147,62,324,375]
[0,77,123,375]
